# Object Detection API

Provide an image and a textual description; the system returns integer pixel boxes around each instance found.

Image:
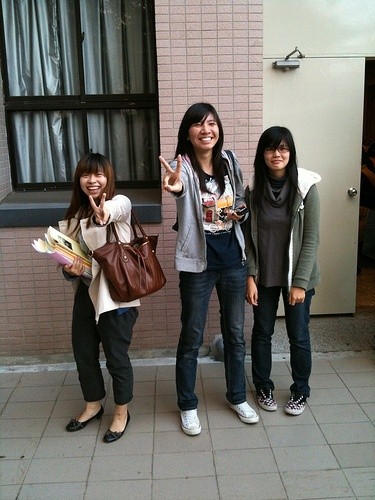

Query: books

[30,226,93,279]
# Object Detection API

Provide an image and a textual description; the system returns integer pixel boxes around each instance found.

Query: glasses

[265,146,291,154]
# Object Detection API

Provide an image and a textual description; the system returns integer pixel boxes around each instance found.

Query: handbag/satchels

[94,207,167,303]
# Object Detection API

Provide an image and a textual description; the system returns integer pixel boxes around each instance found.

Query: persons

[159,102,259,436]
[56,152,141,442]
[358,129,375,274]
[240,126,321,415]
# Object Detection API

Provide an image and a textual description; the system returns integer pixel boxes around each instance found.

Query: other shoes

[65,402,104,432]
[103,409,131,442]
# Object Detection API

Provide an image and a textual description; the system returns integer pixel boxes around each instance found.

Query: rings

[298,301,300,303]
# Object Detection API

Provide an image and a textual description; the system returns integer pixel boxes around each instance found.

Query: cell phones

[232,208,248,215]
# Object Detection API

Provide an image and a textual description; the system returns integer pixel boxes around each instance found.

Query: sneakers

[256,388,277,411]
[180,408,201,436]
[284,391,307,414]
[227,398,260,423]
